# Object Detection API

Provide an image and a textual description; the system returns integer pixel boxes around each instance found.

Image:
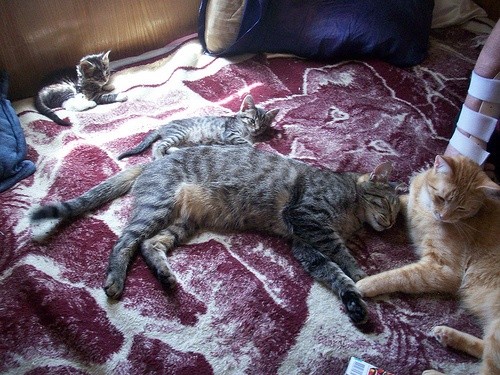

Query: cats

[352,153,500,375]
[30,93,399,326]
[35,51,129,127]
